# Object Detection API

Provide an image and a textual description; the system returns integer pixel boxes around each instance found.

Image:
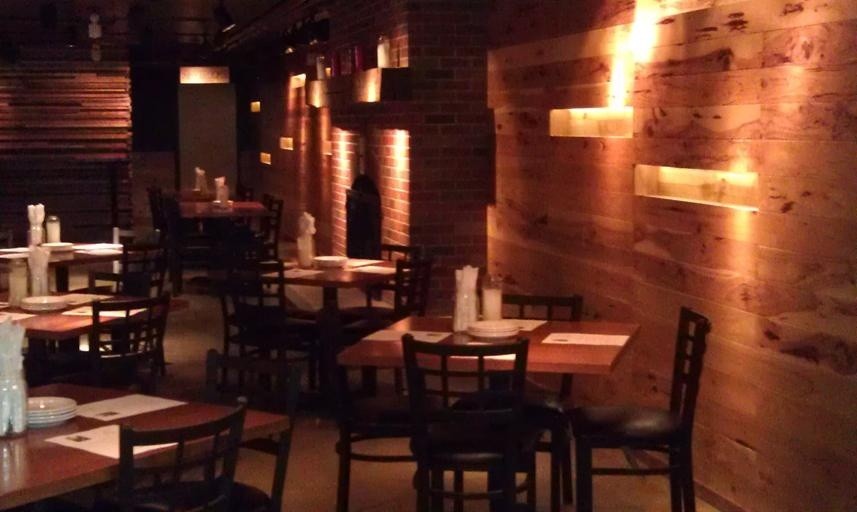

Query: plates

[43,242,74,251]
[212,200,232,205]
[20,297,67,312]
[24,396,80,427]
[315,256,349,267]
[466,323,520,338]
[0,302,10,309]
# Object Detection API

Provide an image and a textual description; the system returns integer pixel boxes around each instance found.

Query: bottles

[452,289,478,333]
[45,216,60,243]
[296,236,312,267]
[27,225,43,246]
[1,371,28,436]
[30,272,49,295]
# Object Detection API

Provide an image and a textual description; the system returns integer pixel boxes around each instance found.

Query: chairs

[148,184,285,297]
[456,293,583,512]
[204,350,301,511]
[118,396,248,512]
[210,237,431,395]
[401,335,546,511]
[574,309,711,511]
[49,293,169,393]
[72,238,169,295]
[321,307,447,512]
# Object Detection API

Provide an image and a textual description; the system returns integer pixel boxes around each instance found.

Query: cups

[8,262,26,307]
[481,275,504,322]
[219,187,229,209]
